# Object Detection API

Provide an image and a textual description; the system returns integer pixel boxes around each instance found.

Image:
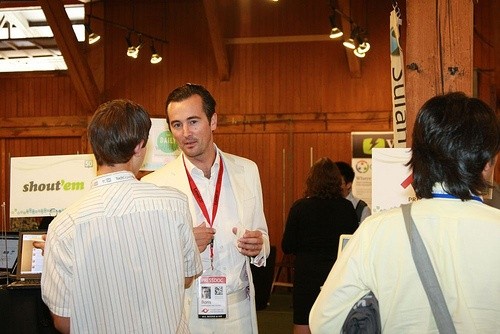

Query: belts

[187,286,247,305]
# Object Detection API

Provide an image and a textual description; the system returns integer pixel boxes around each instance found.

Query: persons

[140,83,270,334]
[284,156,358,334]
[335,161,372,222]
[32,100,203,334]
[309,91,500,334]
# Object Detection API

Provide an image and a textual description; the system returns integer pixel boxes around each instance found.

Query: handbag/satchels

[342,291,381,334]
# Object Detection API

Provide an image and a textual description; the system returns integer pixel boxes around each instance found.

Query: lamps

[126,35,162,64]
[330,15,370,58]
[85,17,100,45]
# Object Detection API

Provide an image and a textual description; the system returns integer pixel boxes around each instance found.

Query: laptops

[7,232,48,290]
[0,237,21,280]
